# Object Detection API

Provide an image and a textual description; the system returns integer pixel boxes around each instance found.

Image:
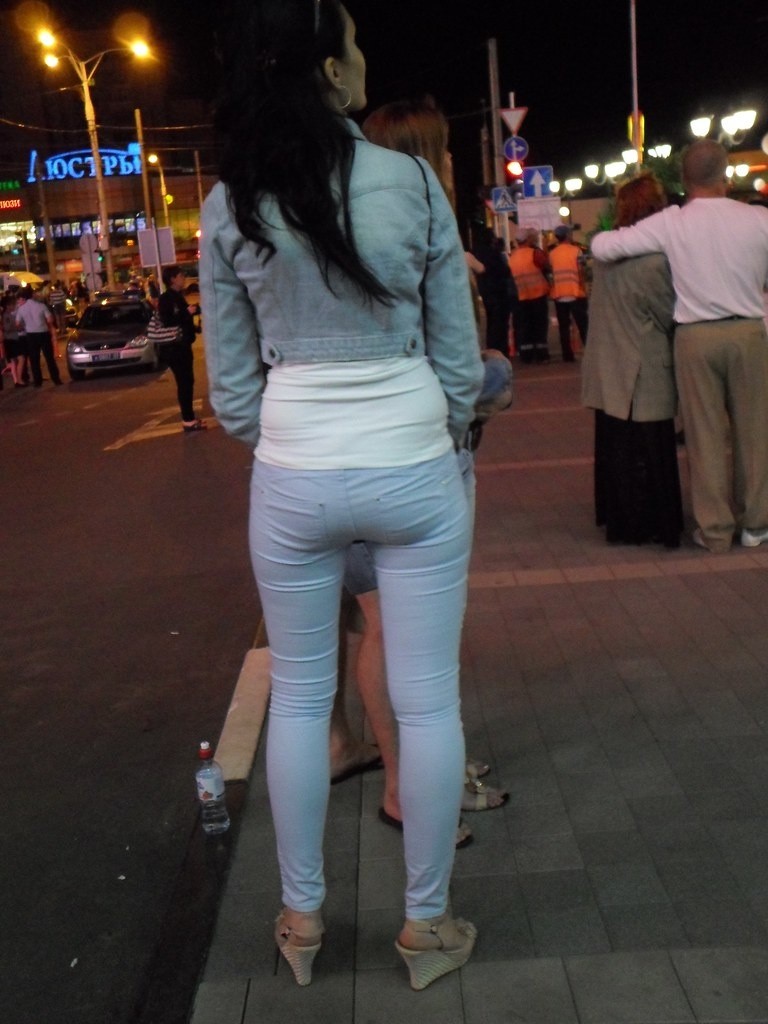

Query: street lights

[585,144,672,205]
[549,178,584,243]
[35,29,175,293]
[689,109,756,182]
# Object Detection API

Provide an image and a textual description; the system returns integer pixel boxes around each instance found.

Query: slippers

[330,741,385,785]
[184,425,208,433]
[464,756,491,781]
[459,780,510,810]
[378,803,474,850]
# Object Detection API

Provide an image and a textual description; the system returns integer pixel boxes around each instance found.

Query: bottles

[195,742,231,833]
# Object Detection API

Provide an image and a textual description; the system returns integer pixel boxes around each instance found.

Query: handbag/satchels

[146,311,183,344]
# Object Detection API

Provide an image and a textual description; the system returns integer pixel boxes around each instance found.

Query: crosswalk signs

[491,186,517,213]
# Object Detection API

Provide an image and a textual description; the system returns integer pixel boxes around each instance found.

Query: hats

[555,226,569,236]
[515,228,530,242]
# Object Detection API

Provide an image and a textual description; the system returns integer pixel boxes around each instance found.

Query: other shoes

[741,528,768,547]
[694,527,707,549]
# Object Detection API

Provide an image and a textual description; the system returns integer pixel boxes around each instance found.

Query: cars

[171,240,202,276]
[65,296,160,380]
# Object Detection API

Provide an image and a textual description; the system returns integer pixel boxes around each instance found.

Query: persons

[492,236,507,255]
[589,144,766,551]
[546,224,593,362]
[507,227,554,365]
[580,172,684,553]
[323,523,472,852]
[475,226,519,361]
[363,98,514,811]
[192,0,486,989]
[522,226,557,364]
[153,265,210,430]
[0,261,160,392]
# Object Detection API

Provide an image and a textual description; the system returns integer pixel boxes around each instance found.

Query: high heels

[273,906,324,984]
[394,917,478,989]
